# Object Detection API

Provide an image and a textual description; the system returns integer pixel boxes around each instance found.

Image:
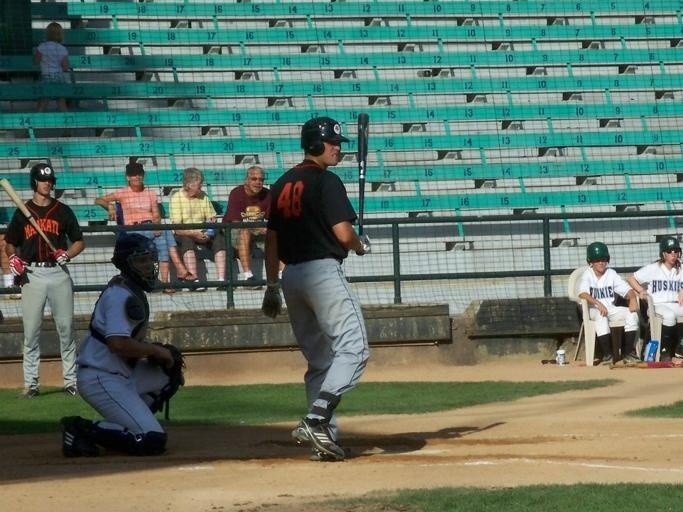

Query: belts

[27,262,56,268]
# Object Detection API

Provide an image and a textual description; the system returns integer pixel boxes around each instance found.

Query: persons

[168,168,233,291]
[22,21,71,137]
[629,237,683,365]
[5,164,84,398]
[60,232,184,456]
[220,166,284,280]
[578,242,641,368]
[93,164,198,292]
[260,117,371,461]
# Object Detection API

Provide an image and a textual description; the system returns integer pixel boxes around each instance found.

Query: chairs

[641,282,683,363]
[568,266,641,366]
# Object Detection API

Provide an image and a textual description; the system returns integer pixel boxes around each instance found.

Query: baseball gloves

[148,340,181,377]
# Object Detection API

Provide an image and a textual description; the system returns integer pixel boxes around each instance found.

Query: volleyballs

[356,113,369,238]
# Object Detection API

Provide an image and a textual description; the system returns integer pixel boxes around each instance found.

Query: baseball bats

[0,178,57,253]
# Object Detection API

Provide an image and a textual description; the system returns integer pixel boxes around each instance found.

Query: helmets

[586,240,610,264]
[109,233,161,293]
[659,236,682,257]
[301,114,351,145]
[30,163,57,182]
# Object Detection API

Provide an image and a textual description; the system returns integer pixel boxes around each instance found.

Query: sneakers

[291,416,346,462]
[61,416,82,457]
[16,385,78,399]
[602,352,641,365]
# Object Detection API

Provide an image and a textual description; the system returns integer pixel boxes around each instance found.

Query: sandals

[179,271,200,283]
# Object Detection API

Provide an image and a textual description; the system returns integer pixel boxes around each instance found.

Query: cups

[556,350,566,367]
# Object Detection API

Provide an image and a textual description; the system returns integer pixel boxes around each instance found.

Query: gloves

[8,252,30,275]
[53,249,71,267]
[261,282,282,320]
